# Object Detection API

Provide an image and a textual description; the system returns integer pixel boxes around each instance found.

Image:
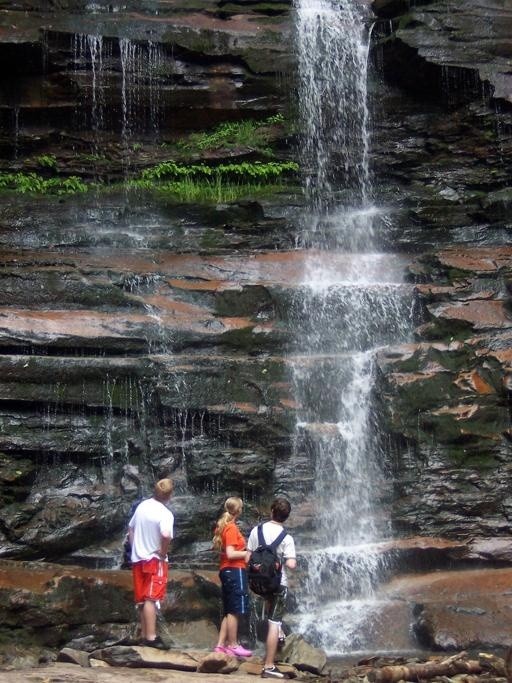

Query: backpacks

[246,524,288,595]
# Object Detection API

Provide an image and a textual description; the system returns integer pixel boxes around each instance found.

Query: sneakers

[213,646,252,656]
[279,637,285,646]
[140,636,168,649]
[261,666,297,678]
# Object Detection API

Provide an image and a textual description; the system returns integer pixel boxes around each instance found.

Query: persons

[128,478,175,650]
[244,497,297,679]
[212,496,253,656]
